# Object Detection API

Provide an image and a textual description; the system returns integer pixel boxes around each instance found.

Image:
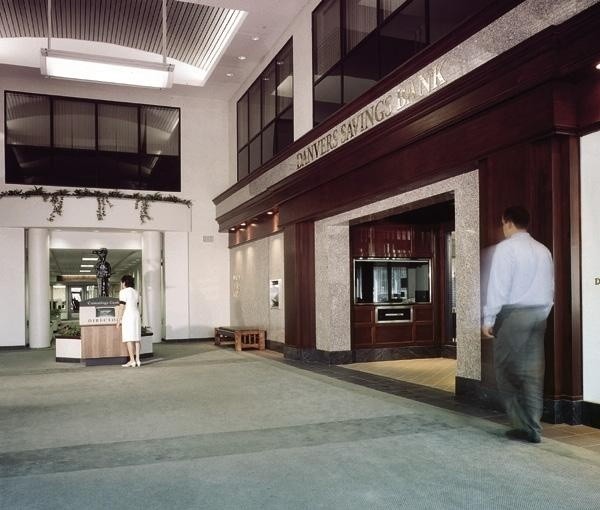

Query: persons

[480,203,556,443]
[116,274,142,367]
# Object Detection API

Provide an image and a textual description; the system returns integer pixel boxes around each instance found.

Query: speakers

[57,275,64,281]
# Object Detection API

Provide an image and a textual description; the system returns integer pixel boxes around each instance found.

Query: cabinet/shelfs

[349,205,442,349]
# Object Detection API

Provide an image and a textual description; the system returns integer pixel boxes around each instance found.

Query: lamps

[42,0,175,90]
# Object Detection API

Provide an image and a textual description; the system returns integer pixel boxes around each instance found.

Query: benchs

[214,325,265,351]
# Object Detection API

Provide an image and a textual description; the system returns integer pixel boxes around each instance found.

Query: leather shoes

[121,359,141,368]
[505,426,542,444]
